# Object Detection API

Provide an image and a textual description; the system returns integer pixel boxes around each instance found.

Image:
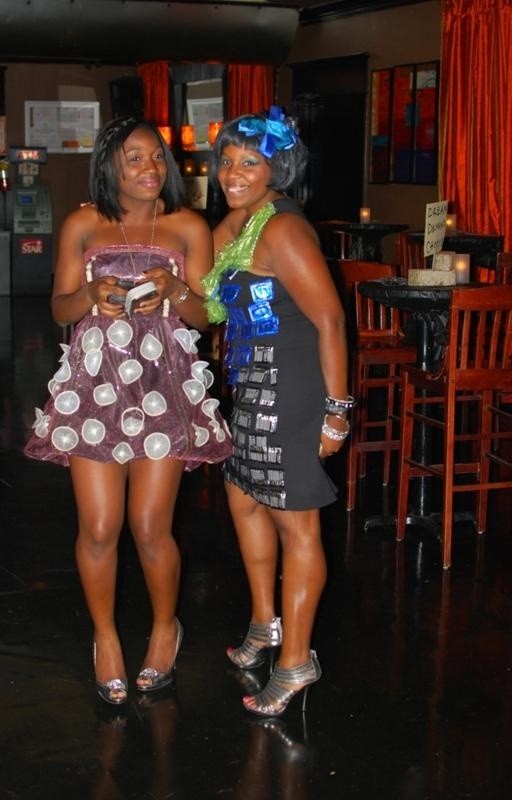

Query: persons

[211,105,358,719]
[51,115,234,706]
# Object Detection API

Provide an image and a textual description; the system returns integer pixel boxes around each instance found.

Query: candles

[358,208,370,224]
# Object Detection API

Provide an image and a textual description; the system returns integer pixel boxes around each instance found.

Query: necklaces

[117,198,158,283]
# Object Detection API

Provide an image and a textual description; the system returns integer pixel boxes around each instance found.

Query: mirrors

[171,78,226,153]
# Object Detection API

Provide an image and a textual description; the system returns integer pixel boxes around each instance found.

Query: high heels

[227,617,282,674]
[257,719,309,764]
[243,650,321,718]
[94,639,128,705]
[136,616,184,691]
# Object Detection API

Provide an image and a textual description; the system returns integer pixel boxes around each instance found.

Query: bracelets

[170,285,192,304]
[324,396,356,415]
[320,416,352,442]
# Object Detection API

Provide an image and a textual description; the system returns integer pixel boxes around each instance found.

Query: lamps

[157,123,225,176]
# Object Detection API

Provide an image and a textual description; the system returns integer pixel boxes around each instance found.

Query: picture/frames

[186,97,225,150]
[24,99,101,157]
